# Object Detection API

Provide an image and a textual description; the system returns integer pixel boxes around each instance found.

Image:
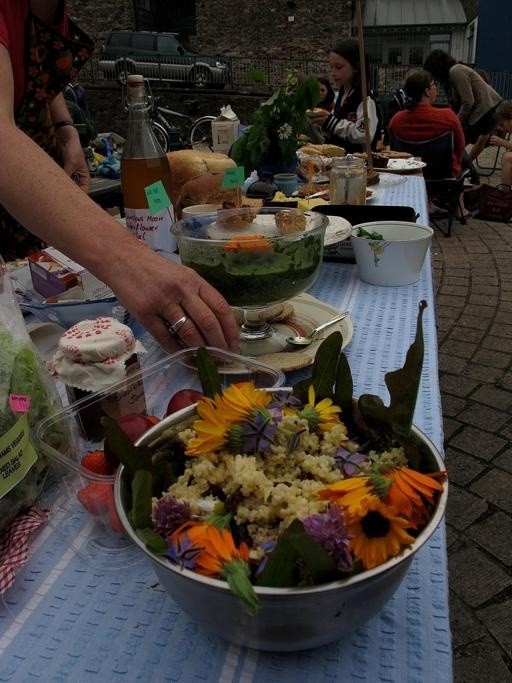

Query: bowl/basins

[0,251,181,342]
[182,203,224,219]
[117,384,448,656]
[349,220,435,288]
[262,195,307,209]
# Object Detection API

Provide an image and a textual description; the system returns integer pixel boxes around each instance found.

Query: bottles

[118,73,178,257]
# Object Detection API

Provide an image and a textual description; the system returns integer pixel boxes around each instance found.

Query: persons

[309,76,335,145]
[60,74,100,142]
[309,37,379,154]
[382,48,512,224]
[1,0,239,368]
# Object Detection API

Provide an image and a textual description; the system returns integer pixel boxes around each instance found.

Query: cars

[379,65,421,92]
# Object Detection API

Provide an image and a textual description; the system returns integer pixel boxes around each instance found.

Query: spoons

[284,309,349,346]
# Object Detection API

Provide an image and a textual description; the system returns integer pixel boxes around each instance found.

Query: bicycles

[144,95,218,154]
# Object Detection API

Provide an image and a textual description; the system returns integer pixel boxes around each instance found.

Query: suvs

[99,28,230,89]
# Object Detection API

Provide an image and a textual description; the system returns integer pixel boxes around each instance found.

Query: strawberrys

[73,448,120,530]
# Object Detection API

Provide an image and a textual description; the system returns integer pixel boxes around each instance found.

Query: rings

[168,315,189,336]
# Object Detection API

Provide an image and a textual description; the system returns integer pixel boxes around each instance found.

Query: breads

[297,143,346,158]
[354,151,389,167]
[381,150,413,158]
[163,149,262,223]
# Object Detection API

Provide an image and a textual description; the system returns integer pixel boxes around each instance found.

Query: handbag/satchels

[479,182,512,222]
[311,205,419,263]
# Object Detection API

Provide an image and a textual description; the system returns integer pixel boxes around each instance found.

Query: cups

[330,157,368,205]
[274,173,298,195]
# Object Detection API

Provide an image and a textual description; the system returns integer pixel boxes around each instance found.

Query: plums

[167,389,205,412]
[105,412,162,470]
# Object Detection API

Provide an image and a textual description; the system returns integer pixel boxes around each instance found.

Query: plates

[368,172,410,188]
[205,212,354,249]
[374,159,426,170]
[363,188,375,199]
[173,299,351,373]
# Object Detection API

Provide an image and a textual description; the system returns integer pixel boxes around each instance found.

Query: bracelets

[54,120,75,132]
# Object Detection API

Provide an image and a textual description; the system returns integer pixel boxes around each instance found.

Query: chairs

[386,130,470,237]
